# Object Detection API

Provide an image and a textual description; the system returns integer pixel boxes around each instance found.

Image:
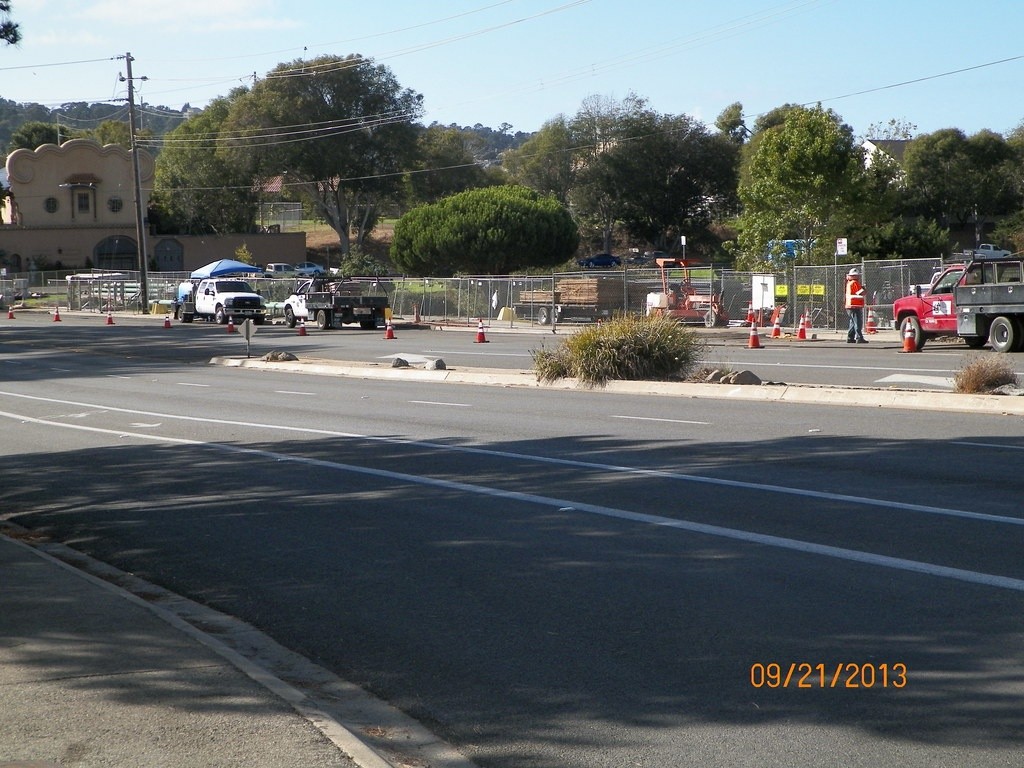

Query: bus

[768,238,818,264]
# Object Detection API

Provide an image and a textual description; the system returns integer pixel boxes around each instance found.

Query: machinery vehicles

[512,258,729,328]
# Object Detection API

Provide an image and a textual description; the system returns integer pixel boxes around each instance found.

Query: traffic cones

[53,306,62,322]
[105,309,115,325]
[740,302,923,352]
[6,303,16,319]
[473,319,490,343]
[226,315,237,332]
[382,318,398,339]
[296,316,309,336]
[413,305,421,323]
[162,311,173,328]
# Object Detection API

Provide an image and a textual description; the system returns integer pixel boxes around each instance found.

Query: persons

[845,268,870,343]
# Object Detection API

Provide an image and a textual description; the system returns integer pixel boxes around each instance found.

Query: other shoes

[847,338,856,343]
[857,338,869,344]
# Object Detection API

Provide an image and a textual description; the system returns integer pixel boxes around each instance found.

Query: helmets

[849,268,862,275]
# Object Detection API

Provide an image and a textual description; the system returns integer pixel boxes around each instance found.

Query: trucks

[170,278,266,325]
[892,249,1024,352]
[283,277,388,330]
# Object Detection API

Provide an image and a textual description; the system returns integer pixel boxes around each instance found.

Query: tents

[189,258,262,280]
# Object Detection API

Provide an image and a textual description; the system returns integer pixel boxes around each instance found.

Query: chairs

[668,283,684,299]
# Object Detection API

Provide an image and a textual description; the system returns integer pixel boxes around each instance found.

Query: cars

[624,251,667,264]
[578,254,621,268]
[294,262,326,277]
[266,263,297,277]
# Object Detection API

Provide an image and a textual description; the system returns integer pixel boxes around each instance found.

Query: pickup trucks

[909,271,965,296]
[963,244,1011,259]
